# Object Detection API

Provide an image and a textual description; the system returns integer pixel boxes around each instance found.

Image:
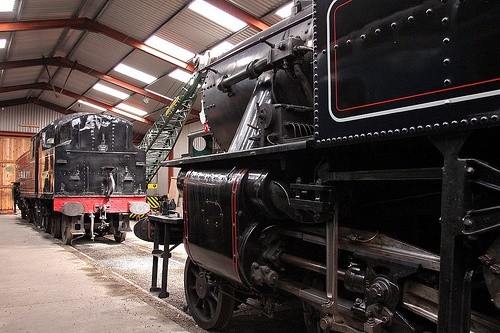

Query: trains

[140,0,500,333]
[11,111,148,248]
